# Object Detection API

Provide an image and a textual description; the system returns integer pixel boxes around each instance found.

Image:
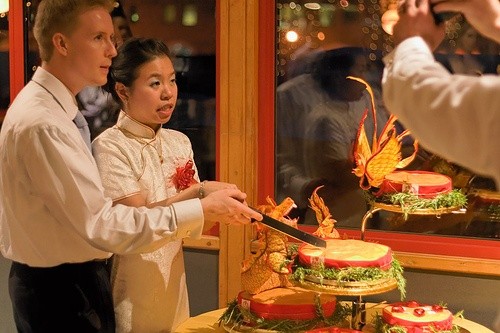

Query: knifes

[232,196,326,248]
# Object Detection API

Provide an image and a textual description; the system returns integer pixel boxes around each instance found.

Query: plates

[369,200,462,215]
[293,276,400,295]
[221,315,287,333]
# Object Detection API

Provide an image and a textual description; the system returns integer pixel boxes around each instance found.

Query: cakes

[284,237,395,284]
[378,300,456,333]
[371,169,457,207]
[236,287,336,326]
[468,186,500,218]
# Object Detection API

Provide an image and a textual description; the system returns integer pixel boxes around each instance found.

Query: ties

[74,111,92,155]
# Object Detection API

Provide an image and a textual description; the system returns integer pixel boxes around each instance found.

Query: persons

[276,24,390,204]
[303,46,370,231]
[0,0,263,333]
[446,20,491,76]
[381,1,500,180]
[78,11,130,129]
[91,37,248,333]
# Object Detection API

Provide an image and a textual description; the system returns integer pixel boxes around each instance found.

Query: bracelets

[198,180,208,199]
